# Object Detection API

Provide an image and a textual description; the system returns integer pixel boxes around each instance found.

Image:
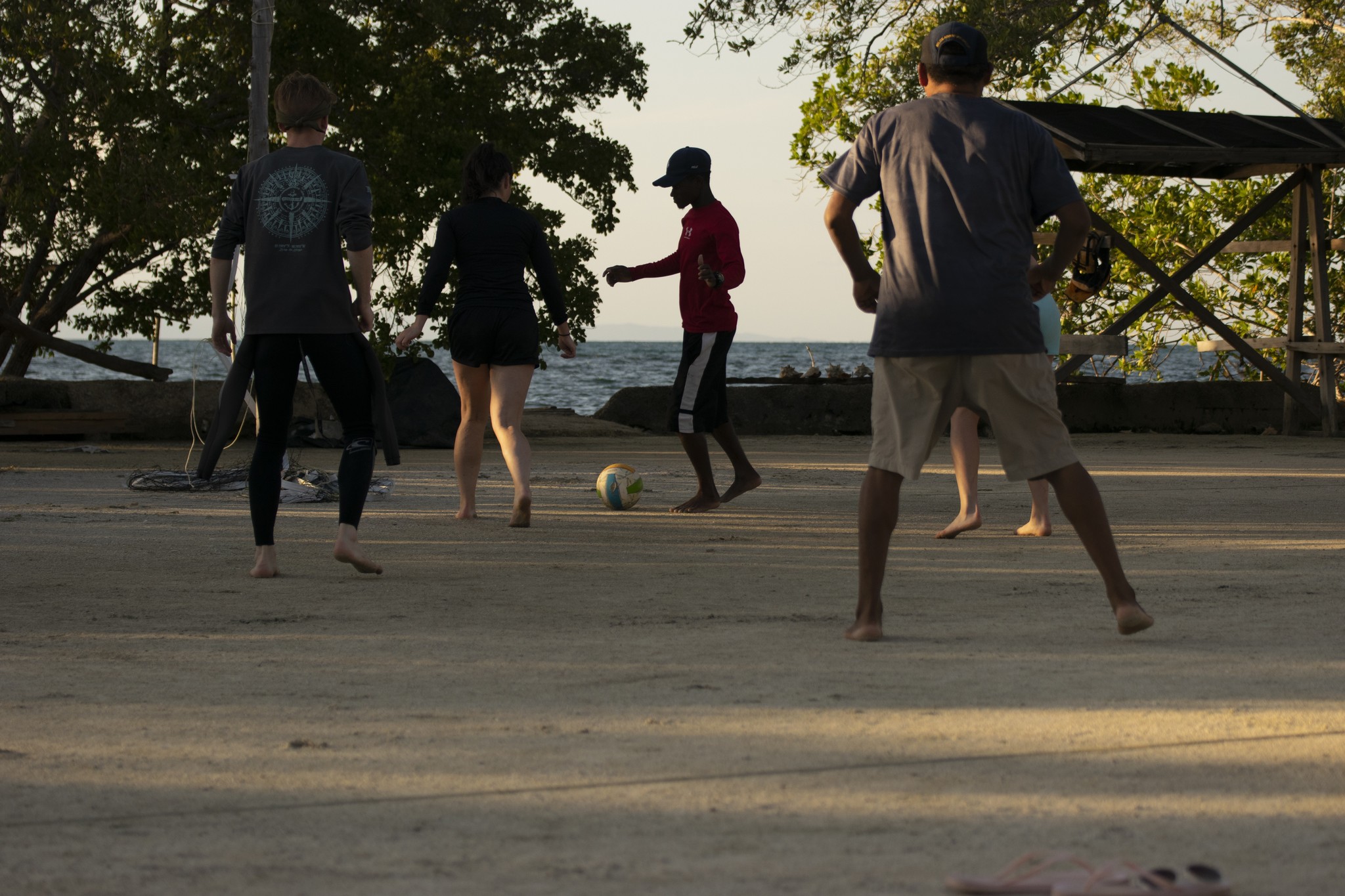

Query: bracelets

[558,332,570,336]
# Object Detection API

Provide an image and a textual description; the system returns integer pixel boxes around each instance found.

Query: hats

[652,146,712,188]
[920,21,988,65]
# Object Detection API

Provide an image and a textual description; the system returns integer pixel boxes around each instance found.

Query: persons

[394,142,576,527]
[932,255,1061,537]
[603,146,762,513]
[208,72,384,578]
[818,22,1156,642]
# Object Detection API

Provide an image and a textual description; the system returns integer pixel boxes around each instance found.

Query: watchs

[711,271,724,289]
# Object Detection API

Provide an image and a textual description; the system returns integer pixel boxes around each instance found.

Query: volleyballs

[595,464,644,510]
